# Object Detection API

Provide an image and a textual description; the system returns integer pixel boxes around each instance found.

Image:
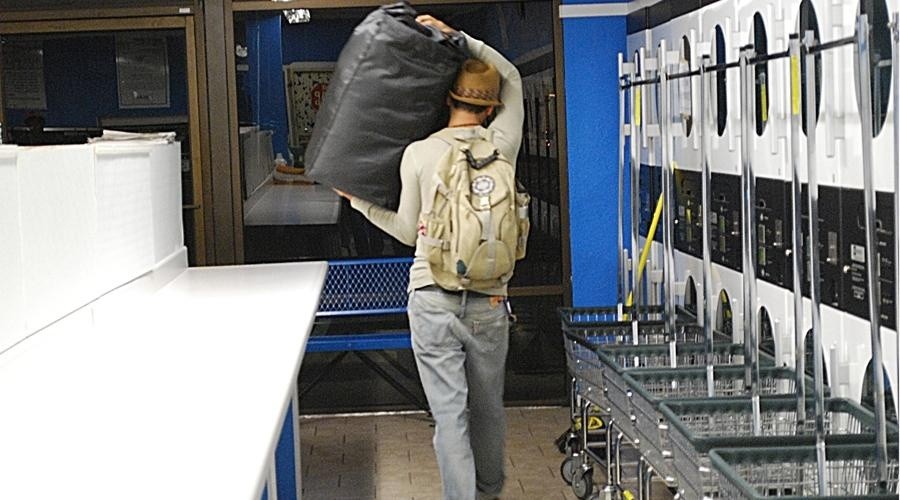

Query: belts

[415,285,495,298]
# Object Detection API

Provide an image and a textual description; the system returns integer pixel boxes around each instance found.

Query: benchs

[272,256,430,417]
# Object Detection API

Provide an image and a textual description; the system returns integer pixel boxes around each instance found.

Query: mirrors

[231,2,564,288]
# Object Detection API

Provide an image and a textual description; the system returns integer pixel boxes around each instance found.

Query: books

[87,126,179,147]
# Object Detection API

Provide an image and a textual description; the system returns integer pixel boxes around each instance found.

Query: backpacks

[427,129,529,292]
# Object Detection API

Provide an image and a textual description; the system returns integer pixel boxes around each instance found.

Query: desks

[246,186,343,257]
[0,261,327,500]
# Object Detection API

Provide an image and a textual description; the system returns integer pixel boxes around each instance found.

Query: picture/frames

[283,62,343,147]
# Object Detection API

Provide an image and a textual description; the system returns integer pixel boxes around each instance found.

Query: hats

[448,59,505,107]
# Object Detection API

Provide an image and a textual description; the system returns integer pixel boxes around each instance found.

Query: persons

[331,16,525,500]
